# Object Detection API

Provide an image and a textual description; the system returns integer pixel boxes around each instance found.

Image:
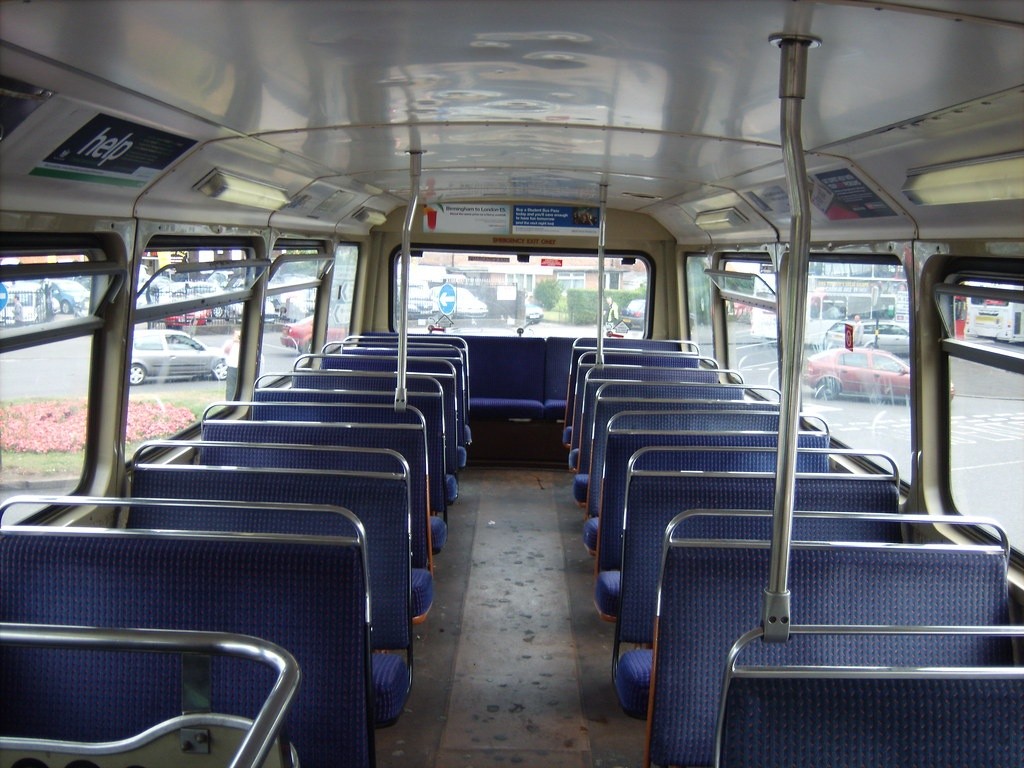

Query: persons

[12,295,22,326]
[223,327,243,402]
[850,316,864,347]
[606,296,619,326]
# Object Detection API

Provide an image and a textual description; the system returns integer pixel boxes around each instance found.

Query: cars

[430,286,489,319]
[128,329,229,386]
[618,299,646,331]
[48,278,87,313]
[163,295,213,328]
[954,295,1004,339]
[803,347,955,405]
[280,311,353,354]
[525,301,544,325]
[396,280,440,320]
[138,273,320,323]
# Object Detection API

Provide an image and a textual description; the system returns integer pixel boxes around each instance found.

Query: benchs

[116,441,415,728]
[251,373,449,556]
[199,401,435,625]
[341,335,472,447]
[291,354,460,507]
[710,624,1024,768]
[583,381,782,556]
[321,341,467,474]
[358,332,545,423]
[574,363,746,510]
[562,338,702,450]
[545,336,679,424]
[590,409,829,624]
[0,494,377,768]
[568,350,719,474]
[641,508,1011,768]
[612,445,901,722]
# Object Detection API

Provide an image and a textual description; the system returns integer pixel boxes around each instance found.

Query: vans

[0,283,46,325]
[823,321,910,357]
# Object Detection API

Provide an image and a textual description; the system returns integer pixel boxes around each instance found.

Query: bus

[963,281,1024,343]
[750,273,910,349]
